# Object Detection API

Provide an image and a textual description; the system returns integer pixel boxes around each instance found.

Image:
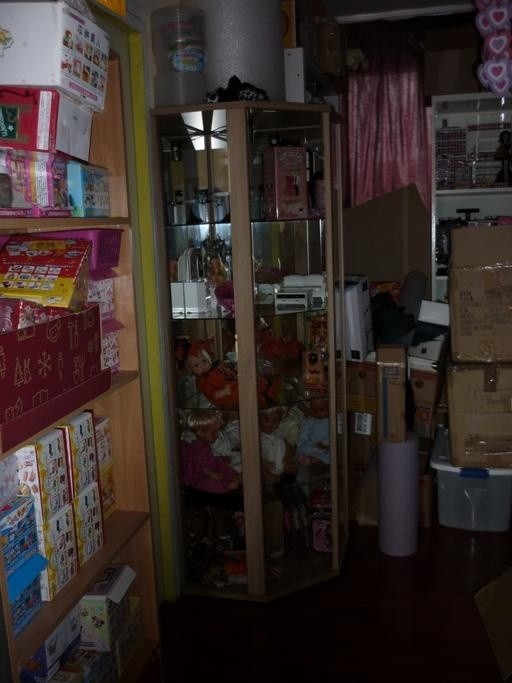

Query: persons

[172,326,331,589]
[178,339,331,532]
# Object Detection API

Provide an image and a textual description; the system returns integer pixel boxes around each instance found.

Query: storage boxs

[396,327,444,433]
[354,344,410,443]
[430,428,511,532]
[446,365,510,466]
[446,226,512,363]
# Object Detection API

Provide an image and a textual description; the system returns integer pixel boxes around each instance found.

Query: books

[466,112,511,189]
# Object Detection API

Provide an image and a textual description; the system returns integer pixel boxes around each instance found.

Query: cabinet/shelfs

[152,100,355,603]
[1,43,167,683]
[428,90,512,303]
